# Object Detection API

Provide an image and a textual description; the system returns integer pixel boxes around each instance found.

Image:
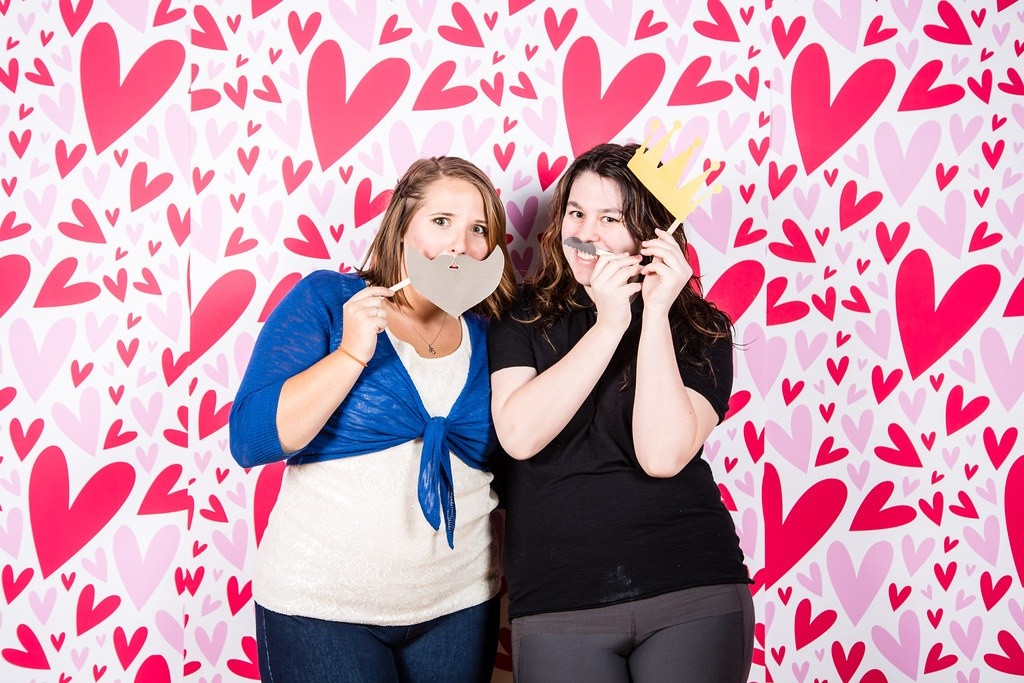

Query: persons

[228,142,755,683]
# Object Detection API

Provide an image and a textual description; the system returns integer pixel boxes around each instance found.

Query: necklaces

[401,310,447,356]
